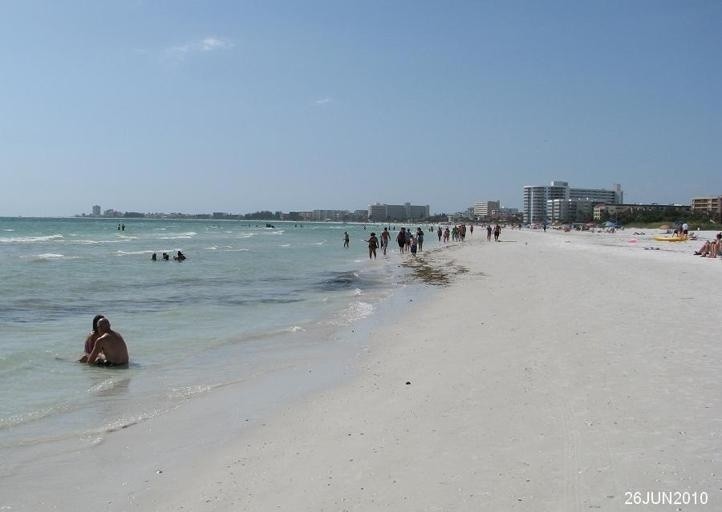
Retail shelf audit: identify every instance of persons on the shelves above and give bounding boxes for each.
[54,317,128,368]
[150,248,188,263]
[122,224,126,231]
[667,221,722,260]
[117,224,121,230]
[84,315,106,356]
[340,223,502,263]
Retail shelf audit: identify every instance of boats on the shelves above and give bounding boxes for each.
[654,231,695,241]
